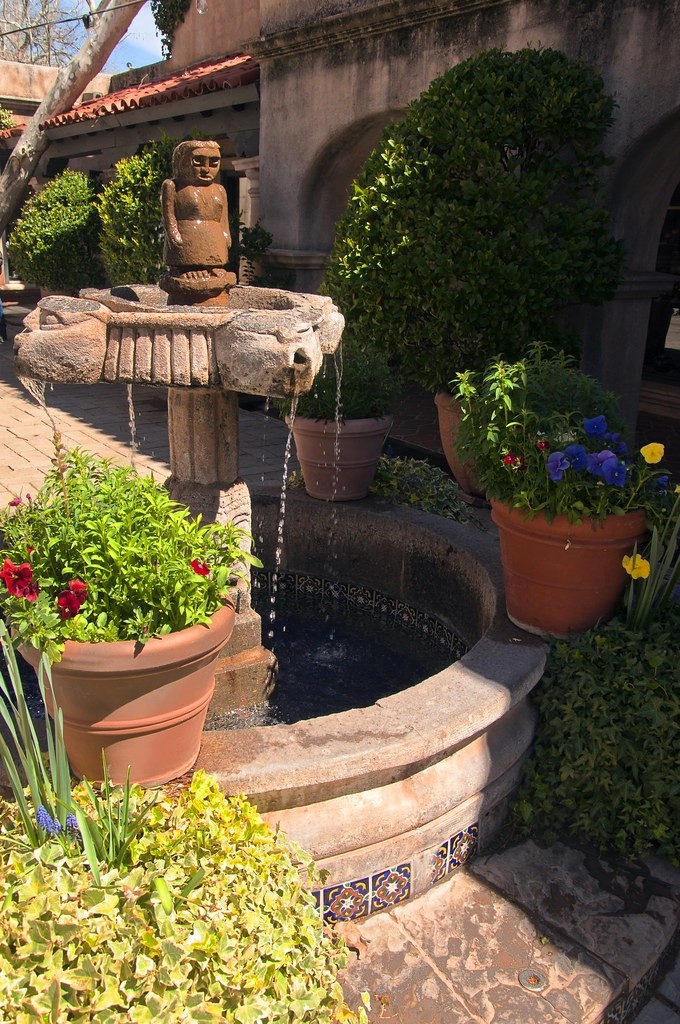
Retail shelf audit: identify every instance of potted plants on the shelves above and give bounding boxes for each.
[391,285,516,499]
[277,355,395,501]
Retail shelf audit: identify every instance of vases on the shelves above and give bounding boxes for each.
[12,595,235,792]
[490,498,645,641]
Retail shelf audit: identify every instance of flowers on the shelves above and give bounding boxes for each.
[0,438,264,672]
[450,354,680,578]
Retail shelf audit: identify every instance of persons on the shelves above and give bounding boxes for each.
[160,140,232,280]
